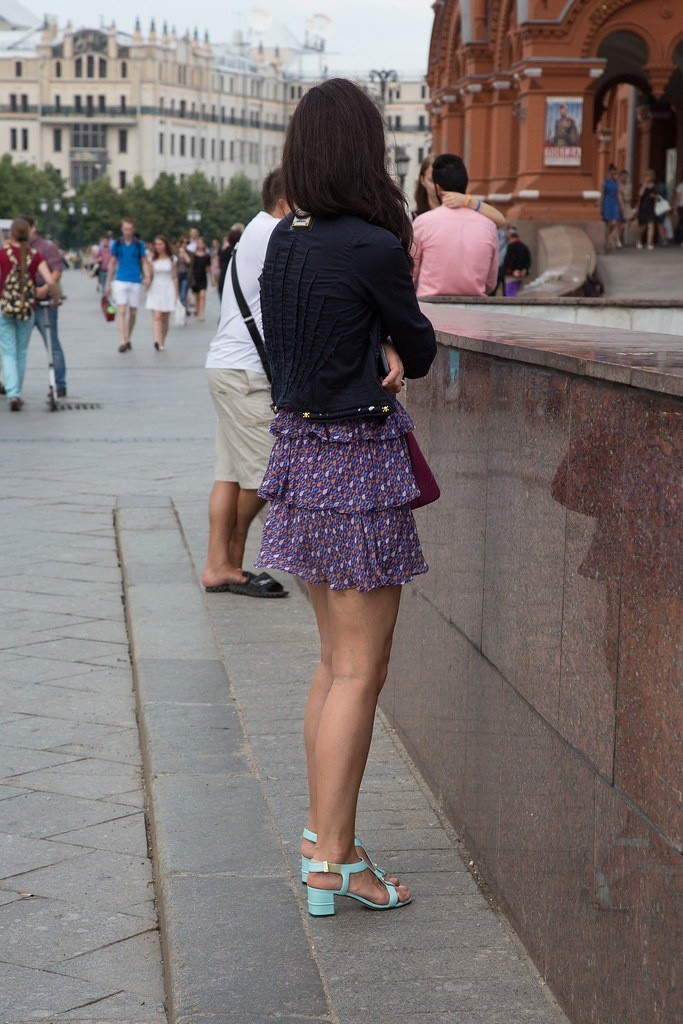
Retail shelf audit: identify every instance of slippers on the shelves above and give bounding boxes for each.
[204,566,292,599]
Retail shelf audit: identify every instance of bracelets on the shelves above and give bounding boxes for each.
[464,193,471,206]
[475,200,481,211]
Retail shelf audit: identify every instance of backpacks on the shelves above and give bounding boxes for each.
[1,242,37,322]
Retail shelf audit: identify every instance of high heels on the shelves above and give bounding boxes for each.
[299,824,397,887]
[307,844,415,916]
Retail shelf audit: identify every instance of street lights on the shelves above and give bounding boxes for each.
[40,197,61,239]
[66,200,89,257]
[185,196,203,245]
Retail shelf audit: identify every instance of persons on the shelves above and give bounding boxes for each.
[256,80,440,920]
[0,215,247,412]
[410,151,532,298]
[202,168,296,599]
[601,163,683,249]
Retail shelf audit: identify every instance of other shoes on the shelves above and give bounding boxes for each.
[638,242,643,248]
[119,341,131,353]
[647,244,654,250]
[10,395,20,411]
[154,341,164,351]
[48,383,67,399]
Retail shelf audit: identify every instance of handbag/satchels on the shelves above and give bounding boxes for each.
[170,298,186,326]
[654,194,671,217]
[664,217,673,239]
[101,293,117,322]
[402,432,441,511]
